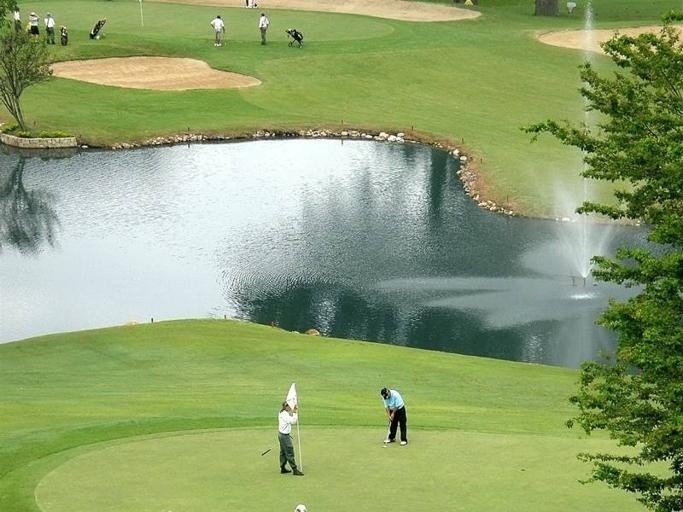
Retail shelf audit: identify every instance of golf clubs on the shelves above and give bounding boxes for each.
[381,421,391,448]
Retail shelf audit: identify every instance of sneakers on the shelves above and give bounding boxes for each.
[280,468,304,475]
[383,438,407,445]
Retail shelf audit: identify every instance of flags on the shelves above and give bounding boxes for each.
[285,382,298,411]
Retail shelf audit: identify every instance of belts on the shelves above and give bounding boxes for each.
[278,431,293,439]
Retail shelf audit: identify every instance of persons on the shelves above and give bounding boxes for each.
[277,401,304,477]
[380,387,409,446]
[43,13,55,44]
[257,12,269,45]
[13,7,22,35]
[210,14,225,48]
[26,11,40,43]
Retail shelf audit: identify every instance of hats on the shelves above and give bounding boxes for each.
[381,387,388,400]
